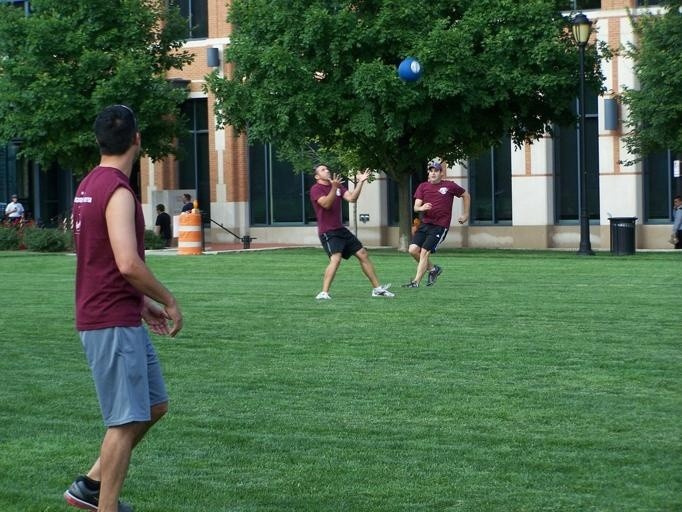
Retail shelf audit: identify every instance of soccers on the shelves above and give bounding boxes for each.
[398,57,421,82]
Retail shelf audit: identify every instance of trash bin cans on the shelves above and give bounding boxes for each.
[609,217,637,254]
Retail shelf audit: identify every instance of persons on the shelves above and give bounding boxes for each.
[400,162,472,289]
[5,193,26,224]
[671,195,682,249]
[181,193,194,212]
[309,164,395,300]
[62,105,184,512]
[153,204,172,246]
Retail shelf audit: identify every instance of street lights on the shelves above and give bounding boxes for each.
[569,9,596,254]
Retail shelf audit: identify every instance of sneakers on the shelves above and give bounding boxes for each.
[62,476,131,512]
[372,287,395,297]
[426,265,442,286]
[316,291,331,299]
[401,281,419,287]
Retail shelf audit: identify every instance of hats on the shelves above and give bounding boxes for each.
[429,162,441,170]
